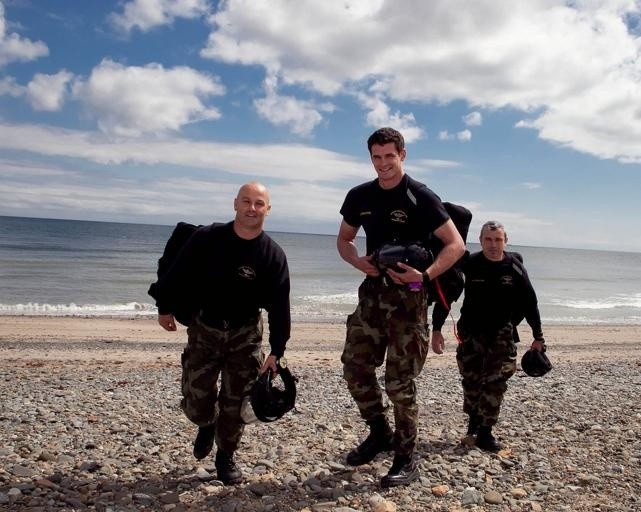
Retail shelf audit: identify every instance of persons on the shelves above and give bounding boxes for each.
[431,219,547,452]
[337,129,466,489]
[155,182,292,486]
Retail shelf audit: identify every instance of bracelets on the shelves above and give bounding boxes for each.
[535,337,545,342]
[422,271,431,287]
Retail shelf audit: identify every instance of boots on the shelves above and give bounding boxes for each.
[467,417,482,433]
[193,425,215,459]
[381,453,420,487]
[477,425,501,452]
[347,421,394,466]
[215,449,242,485]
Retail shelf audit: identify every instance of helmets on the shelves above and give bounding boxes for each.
[239,363,297,425]
[521,349,552,377]
[376,238,434,279]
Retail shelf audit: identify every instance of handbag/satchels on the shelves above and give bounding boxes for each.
[146,222,199,326]
[428,199,472,305]
